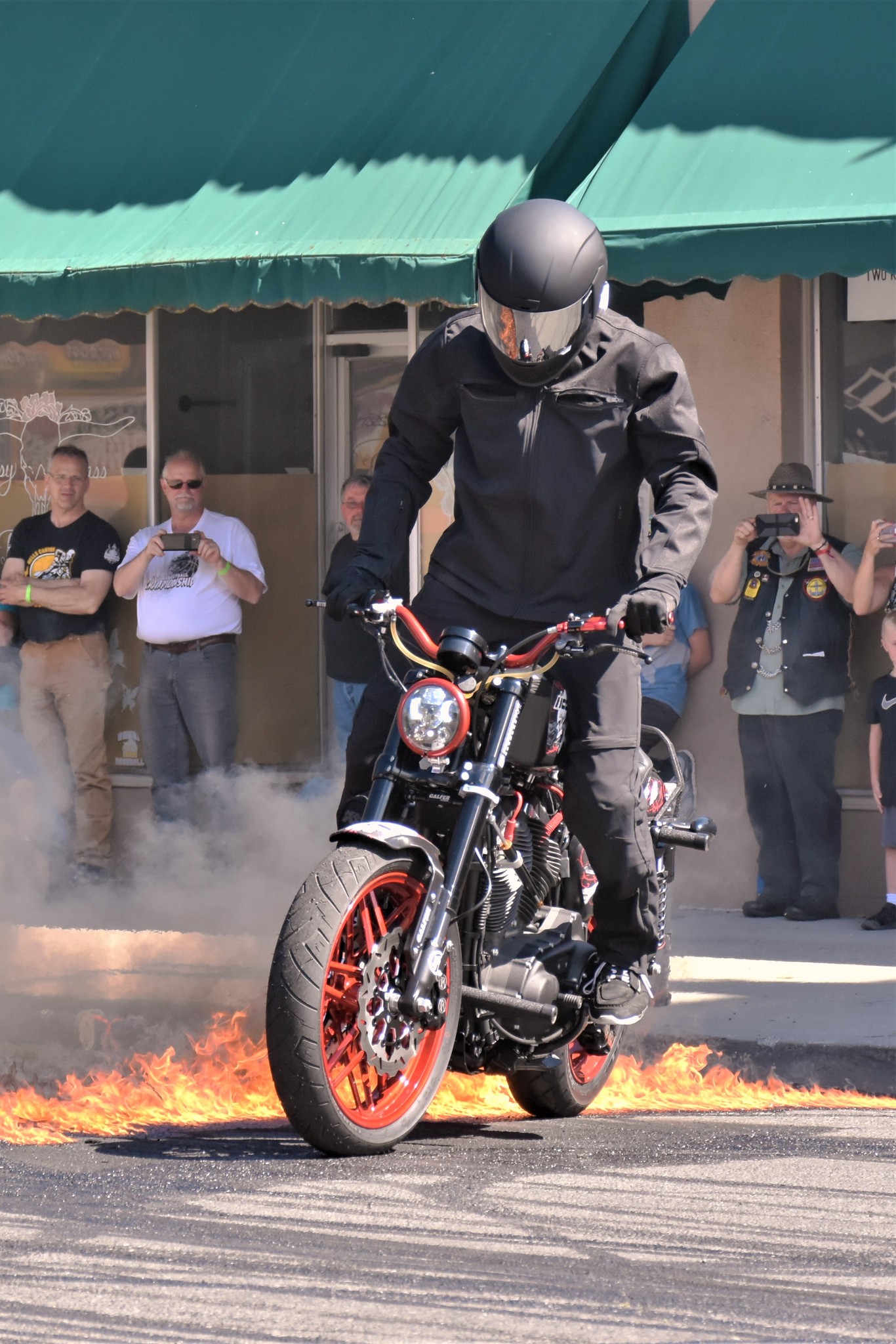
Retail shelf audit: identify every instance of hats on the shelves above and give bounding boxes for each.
[748,463,835,505]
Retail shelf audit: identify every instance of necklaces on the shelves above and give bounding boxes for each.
[764,550,810,577]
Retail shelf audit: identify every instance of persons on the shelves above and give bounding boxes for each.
[321,473,409,751]
[0,446,120,885]
[861,610,896,930]
[329,198,721,1025]
[852,518,896,616]
[708,460,864,921]
[641,582,712,823]
[114,448,268,868]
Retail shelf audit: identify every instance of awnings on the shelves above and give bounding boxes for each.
[0,0,896,323]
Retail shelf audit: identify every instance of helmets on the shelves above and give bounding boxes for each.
[475,198,608,385]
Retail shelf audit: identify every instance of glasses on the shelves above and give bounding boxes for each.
[342,499,366,510]
[47,471,89,486]
[164,476,204,489]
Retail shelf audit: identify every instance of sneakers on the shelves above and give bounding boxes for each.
[583,952,652,1027]
[860,902,896,930]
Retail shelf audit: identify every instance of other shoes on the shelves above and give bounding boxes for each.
[742,892,786,917]
[74,865,101,888]
[784,895,840,921]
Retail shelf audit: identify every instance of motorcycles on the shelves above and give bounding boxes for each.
[261,593,721,1157]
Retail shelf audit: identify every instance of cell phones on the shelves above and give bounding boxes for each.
[878,522,896,543]
[754,513,800,537]
[159,533,199,550]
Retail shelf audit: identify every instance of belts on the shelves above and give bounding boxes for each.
[144,634,237,653]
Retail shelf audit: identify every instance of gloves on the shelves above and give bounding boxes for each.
[608,588,676,644]
[323,568,382,623]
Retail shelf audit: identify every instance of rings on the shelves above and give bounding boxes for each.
[877,537,879,540]
[809,516,813,519]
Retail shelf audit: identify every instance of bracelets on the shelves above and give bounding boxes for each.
[217,561,230,575]
[26,584,32,604]
[814,539,835,559]
